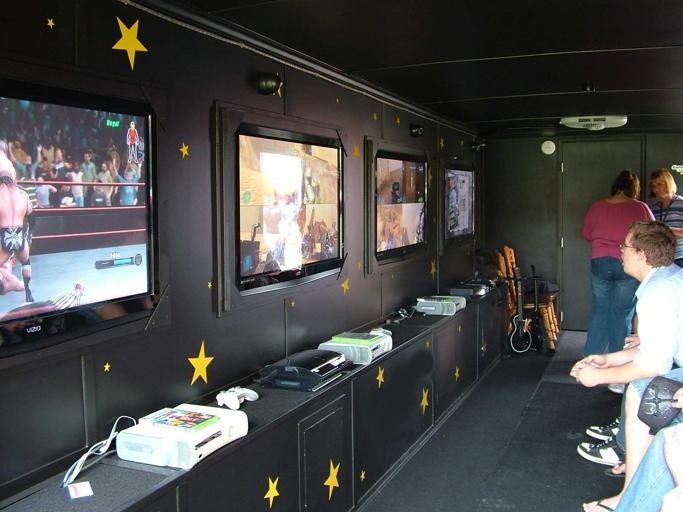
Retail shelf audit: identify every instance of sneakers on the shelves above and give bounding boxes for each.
[606,382,626,394]
[584,415,621,440]
[575,434,626,467]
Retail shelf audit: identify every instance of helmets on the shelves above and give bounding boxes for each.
[0,160,17,182]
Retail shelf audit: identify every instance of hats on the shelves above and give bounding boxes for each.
[637,377,683,435]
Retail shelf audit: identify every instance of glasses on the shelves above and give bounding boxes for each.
[619,241,636,249]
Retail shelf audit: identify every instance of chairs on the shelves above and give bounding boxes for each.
[495,245,559,353]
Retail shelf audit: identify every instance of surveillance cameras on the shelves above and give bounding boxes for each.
[257,72,281,94]
[411,126,424,137]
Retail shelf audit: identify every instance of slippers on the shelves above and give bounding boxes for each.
[595,498,613,512]
[603,463,625,478]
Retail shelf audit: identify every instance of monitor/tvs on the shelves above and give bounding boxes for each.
[234,122,344,291]
[374,149,428,261]
[444,163,476,244]
[0,78,159,359]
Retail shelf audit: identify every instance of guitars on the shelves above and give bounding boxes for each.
[508,265,550,354]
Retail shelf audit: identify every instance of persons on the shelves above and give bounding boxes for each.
[0,97,148,301]
[382,211,409,251]
[262,222,335,276]
[568,216,683,512]
[646,167,683,268]
[447,172,459,231]
[579,169,658,359]
